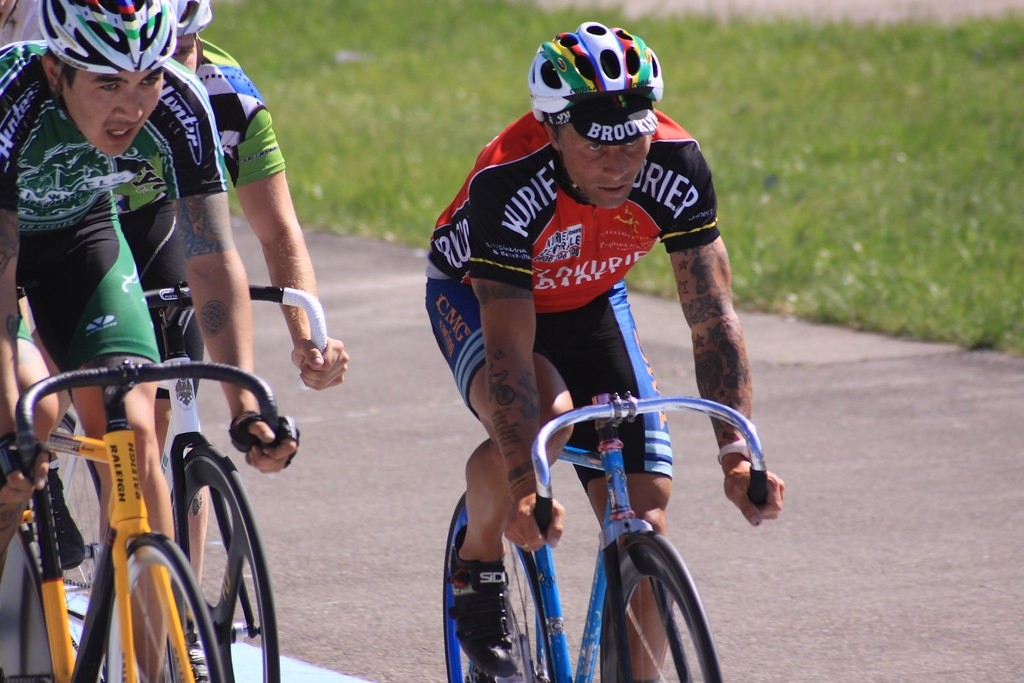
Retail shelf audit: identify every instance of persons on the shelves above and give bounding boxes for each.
[426,22,782,683]
[0,1,349,683]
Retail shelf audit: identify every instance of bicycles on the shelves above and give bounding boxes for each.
[1,285,328,683]
[440,395,769,683]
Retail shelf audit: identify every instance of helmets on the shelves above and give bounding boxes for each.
[528,21,663,145]
[176,0,213,36]
[37,0,177,74]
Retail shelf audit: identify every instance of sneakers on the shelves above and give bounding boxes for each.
[449,525,520,677]
[47,467,86,569]
[185,633,210,683]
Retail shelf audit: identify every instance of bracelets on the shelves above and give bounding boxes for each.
[717,440,752,463]
